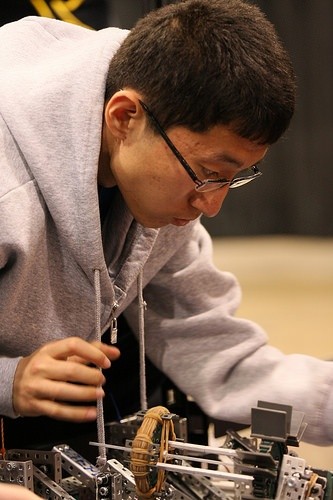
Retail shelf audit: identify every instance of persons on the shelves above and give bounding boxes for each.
[0,0,333,459]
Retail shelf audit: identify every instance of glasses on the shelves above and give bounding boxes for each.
[118,87,263,194]
